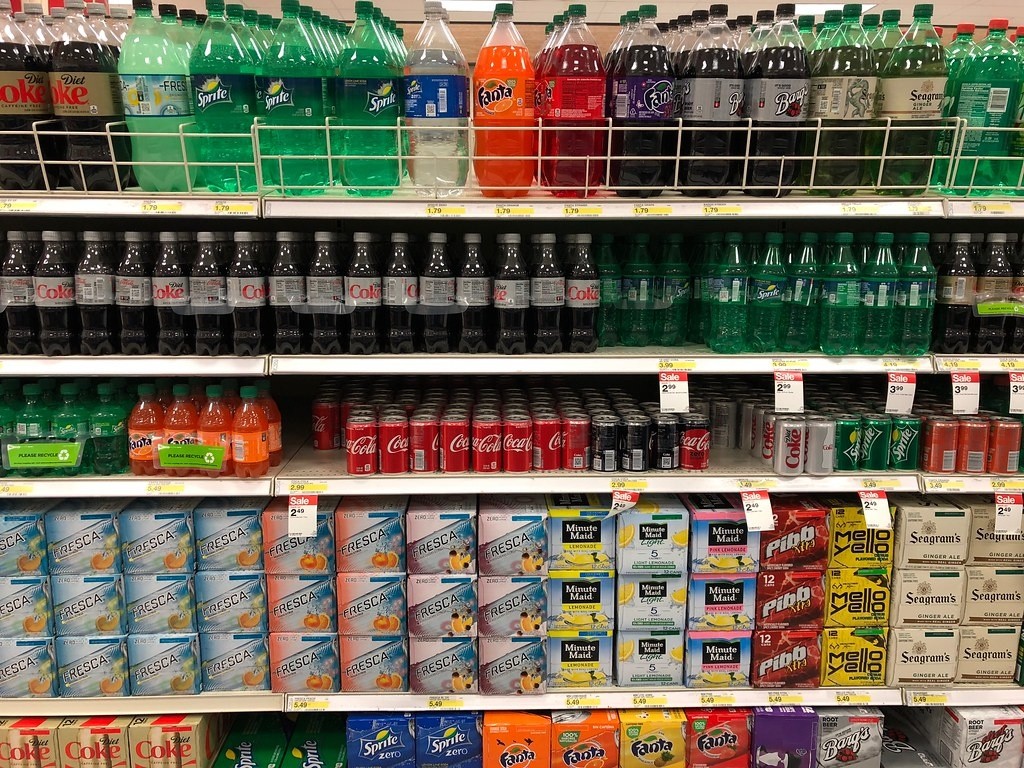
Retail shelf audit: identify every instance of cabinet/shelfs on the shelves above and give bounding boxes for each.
[0,195,1024,716]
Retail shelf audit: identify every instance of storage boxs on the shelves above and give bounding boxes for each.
[0,492,1023,700]
[0,704,1024,768]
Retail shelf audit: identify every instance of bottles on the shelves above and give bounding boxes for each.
[797,4,949,197]
[902,18,1024,197]
[403,0,470,197]
[473,3,536,198]
[0,230,602,356]
[189,0,408,198]
[932,233,1024,355]
[0,0,129,191]
[656,4,811,198]
[117,0,206,193]
[125,377,283,478]
[602,5,675,199]
[595,232,936,354]
[0,377,139,478]
[532,4,607,199]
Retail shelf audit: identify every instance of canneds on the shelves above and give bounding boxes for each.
[313,374,1024,477]
